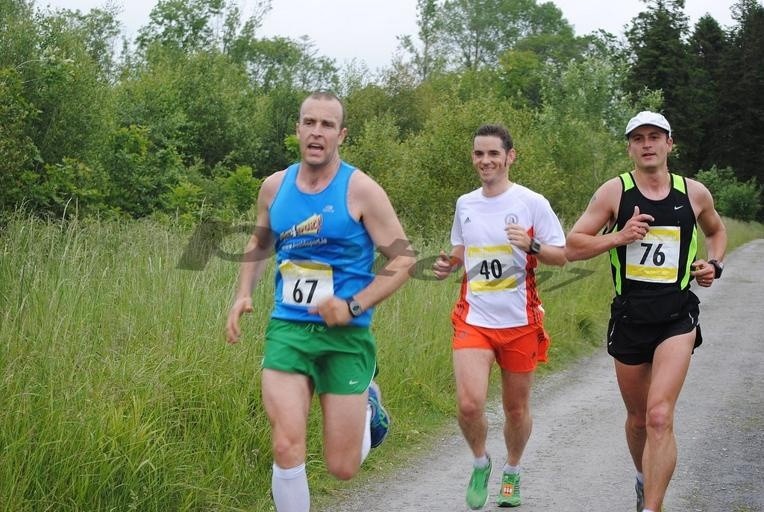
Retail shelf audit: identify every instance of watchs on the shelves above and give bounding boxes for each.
[526,237,540,255]
[708,260,724,279]
[345,296,362,317]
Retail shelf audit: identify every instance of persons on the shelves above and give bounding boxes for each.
[565,111,727,512]
[433,125,568,510]
[225,92,417,512]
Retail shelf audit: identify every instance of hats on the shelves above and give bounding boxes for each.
[625,111,671,137]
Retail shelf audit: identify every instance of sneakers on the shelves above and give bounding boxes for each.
[368,381,390,448]
[635,477,663,512]
[496,461,521,507]
[467,456,492,510]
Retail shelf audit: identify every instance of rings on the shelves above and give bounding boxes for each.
[702,280,705,283]
